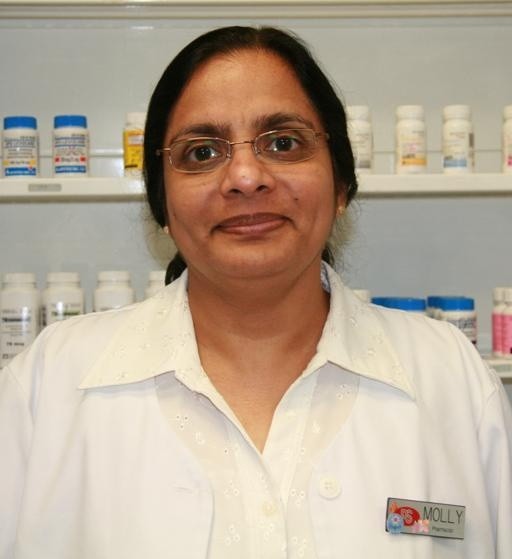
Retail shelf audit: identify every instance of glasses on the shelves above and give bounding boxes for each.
[151,125,331,176]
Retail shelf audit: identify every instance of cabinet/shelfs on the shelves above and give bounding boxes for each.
[0,173,510,381]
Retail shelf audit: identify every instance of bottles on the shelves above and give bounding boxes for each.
[370,296,478,350]
[3,115,40,177]
[145,270,168,301]
[51,114,89,177]
[1,271,41,366]
[42,271,86,329]
[441,104,475,175]
[490,286,512,361]
[345,105,376,175]
[502,106,511,172]
[393,105,427,175]
[93,270,133,312]
[121,111,146,180]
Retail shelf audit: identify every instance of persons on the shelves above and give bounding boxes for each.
[0,24,511,559]
